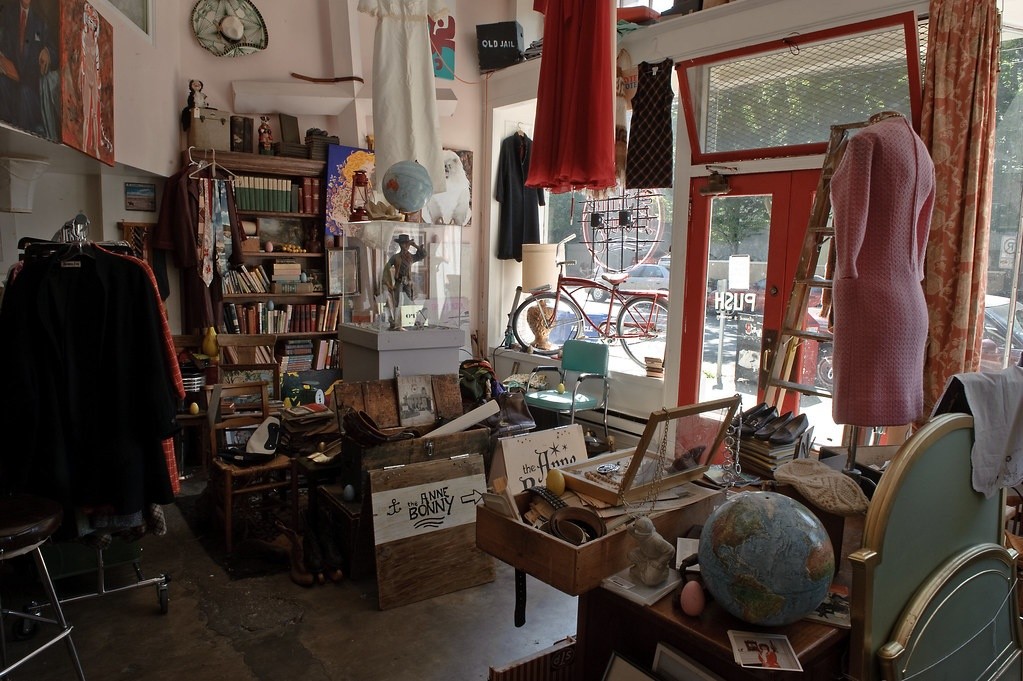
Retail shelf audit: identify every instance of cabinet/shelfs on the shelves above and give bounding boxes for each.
[181,148,344,408]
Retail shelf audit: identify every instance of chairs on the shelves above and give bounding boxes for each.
[524,339,610,438]
[203,380,299,557]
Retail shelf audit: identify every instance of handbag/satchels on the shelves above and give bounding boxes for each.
[479,387,536,436]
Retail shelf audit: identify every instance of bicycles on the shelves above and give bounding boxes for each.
[512,235,668,371]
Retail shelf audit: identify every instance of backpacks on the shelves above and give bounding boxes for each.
[458,357,497,403]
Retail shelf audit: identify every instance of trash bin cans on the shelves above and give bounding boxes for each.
[735,313,763,382]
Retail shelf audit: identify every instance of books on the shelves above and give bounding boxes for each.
[208,176,342,371]
[728,433,807,478]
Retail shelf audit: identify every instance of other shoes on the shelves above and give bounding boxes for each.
[769,413,808,445]
[728,401,768,429]
[737,406,779,436]
[754,410,794,440]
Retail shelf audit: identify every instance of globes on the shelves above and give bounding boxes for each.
[698,491,836,626]
[382,160,433,222]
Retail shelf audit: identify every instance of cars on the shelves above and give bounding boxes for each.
[708,272,826,328]
[815,294,1023,391]
[584,264,671,303]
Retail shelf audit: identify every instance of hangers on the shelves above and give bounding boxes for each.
[517,122,525,137]
[627,36,681,76]
[165,146,239,181]
[18,217,135,265]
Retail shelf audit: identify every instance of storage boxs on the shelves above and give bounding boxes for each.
[475,393,921,681]
[305,135,340,161]
[188,107,230,152]
[273,142,310,158]
[617,5,661,22]
[476,478,728,597]
[475,21,525,73]
[315,417,494,612]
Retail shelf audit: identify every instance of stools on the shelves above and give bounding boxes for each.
[0,492,86,681]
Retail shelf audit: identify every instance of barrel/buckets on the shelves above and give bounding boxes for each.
[175,360,208,414]
[523,243,556,293]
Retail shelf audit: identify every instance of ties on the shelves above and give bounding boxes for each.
[196,178,233,288]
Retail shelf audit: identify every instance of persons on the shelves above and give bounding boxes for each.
[756,643,780,668]
[383,234,427,330]
[830,110,936,426]
[628,516,675,586]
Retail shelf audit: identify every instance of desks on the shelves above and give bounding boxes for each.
[577,492,866,681]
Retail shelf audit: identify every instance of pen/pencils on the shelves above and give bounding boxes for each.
[419,310,426,320]
[807,433,810,453]
[809,436,816,451]
[802,442,806,459]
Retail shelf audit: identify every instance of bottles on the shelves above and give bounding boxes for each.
[287,474,309,511]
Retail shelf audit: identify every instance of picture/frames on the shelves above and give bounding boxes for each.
[124,182,156,211]
[384,230,427,267]
[325,246,361,298]
[382,269,430,300]
[218,363,281,401]
